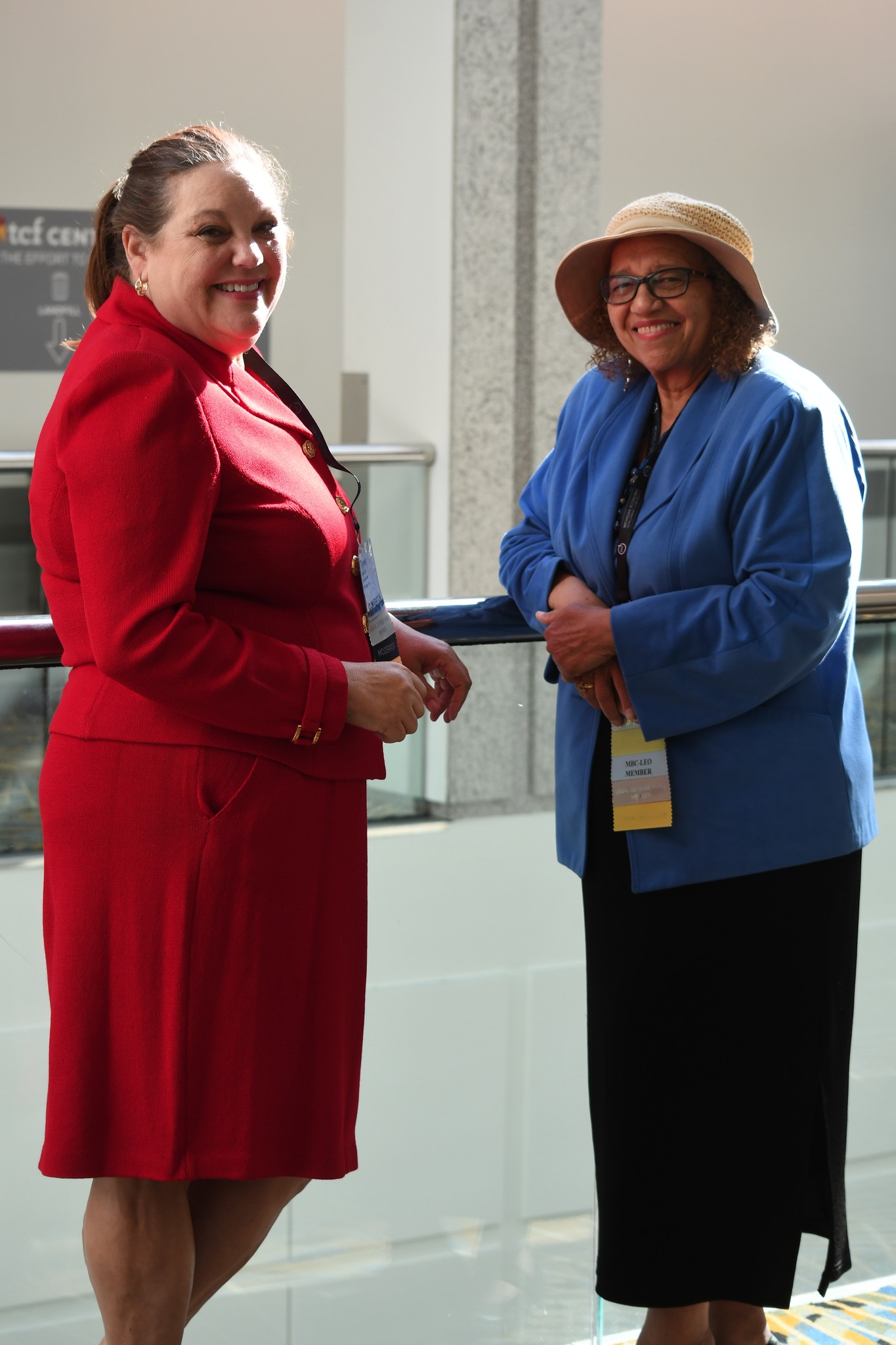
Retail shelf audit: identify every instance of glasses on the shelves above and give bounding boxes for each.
[599,267,722,305]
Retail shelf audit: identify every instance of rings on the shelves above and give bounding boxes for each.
[441,677,447,680]
[578,681,595,693]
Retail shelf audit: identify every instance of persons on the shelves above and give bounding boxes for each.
[26,119,471,1345]
[500,192,880,1342]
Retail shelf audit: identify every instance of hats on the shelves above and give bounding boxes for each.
[554,191,779,351]
